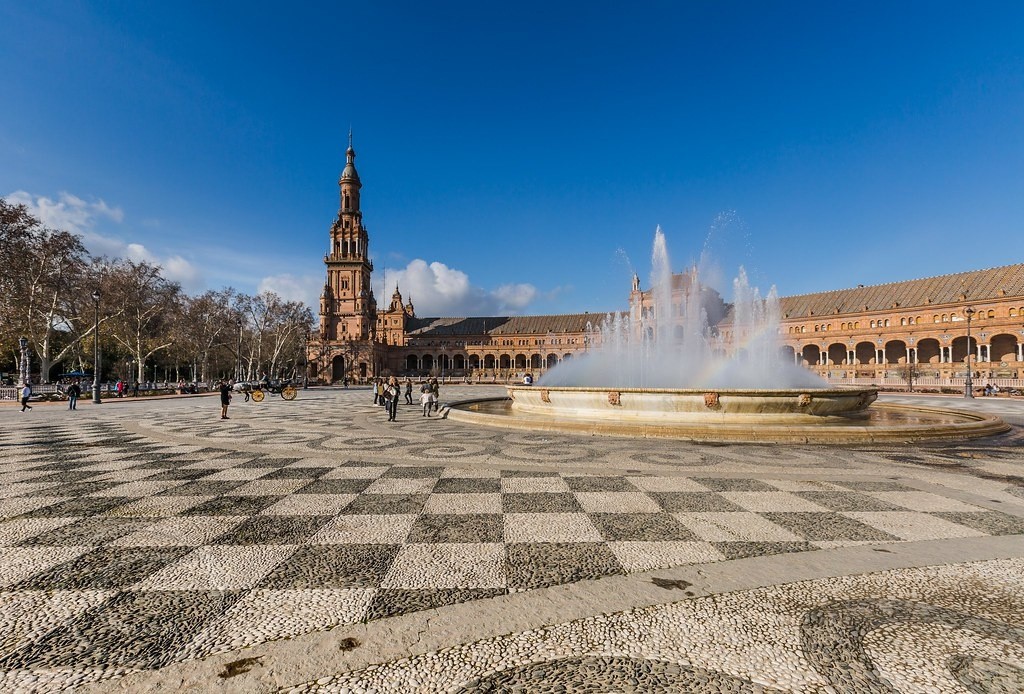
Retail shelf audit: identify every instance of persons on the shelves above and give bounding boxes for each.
[373,374,400,421]
[144,377,234,394]
[521,372,532,386]
[418,375,439,417]
[404,377,413,404]
[985,382,1000,396]
[114,379,139,397]
[19,383,32,413]
[61,377,93,410]
[219,378,232,418]
[260,371,268,381]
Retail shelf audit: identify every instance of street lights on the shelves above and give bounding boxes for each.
[16,334,29,402]
[132,360,136,383]
[583,334,588,353]
[303,334,308,388]
[125,362,129,382]
[236,320,242,383]
[964,308,974,398]
[154,364,157,383]
[91,290,102,403]
[538,344,543,377]
[441,344,446,385]
[189,364,192,382]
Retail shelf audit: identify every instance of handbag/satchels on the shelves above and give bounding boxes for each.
[382,385,393,401]
[229,395,233,400]
[69,390,74,397]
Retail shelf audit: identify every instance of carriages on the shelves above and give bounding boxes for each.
[228,362,298,402]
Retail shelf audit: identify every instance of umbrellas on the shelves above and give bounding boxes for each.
[58,372,91,377]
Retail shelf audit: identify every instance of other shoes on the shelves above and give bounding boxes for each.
[388,417,392,421]
[29,407,32,411]
[392,419,397,422]
[220,416,230,419]
[19,410,24,413]
